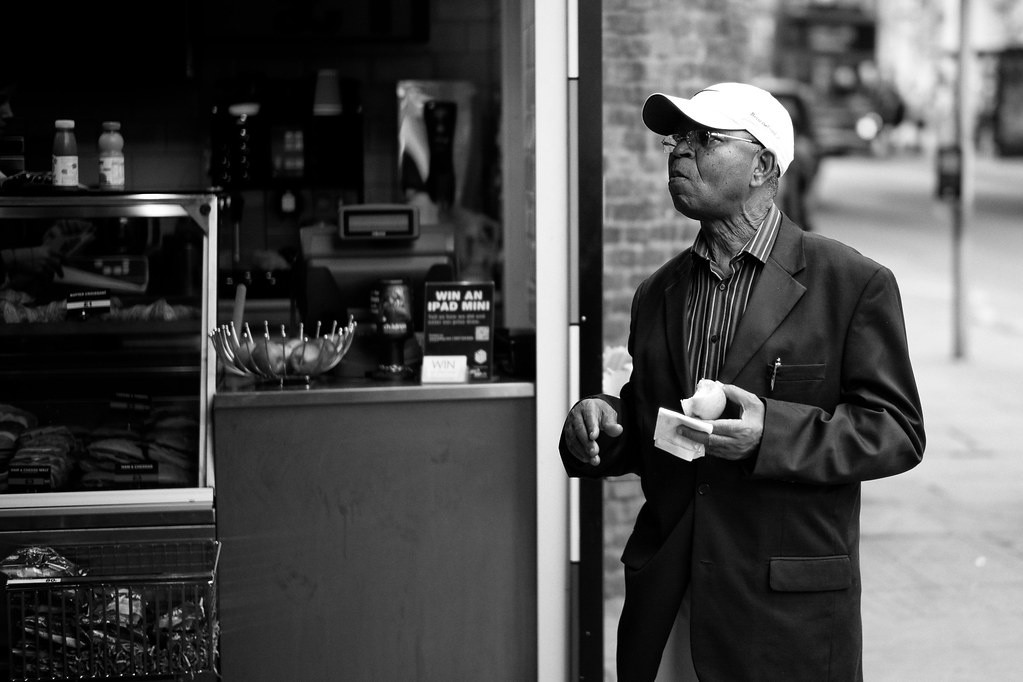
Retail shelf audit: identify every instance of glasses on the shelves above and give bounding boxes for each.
[661,130,765,154]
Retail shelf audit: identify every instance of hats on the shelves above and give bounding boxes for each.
[641,82,794,178]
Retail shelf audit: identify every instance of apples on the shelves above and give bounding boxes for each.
[232,332,346,376]
[680,378,727,420]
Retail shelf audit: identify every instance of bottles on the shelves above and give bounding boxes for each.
[98,122,125,191]
[52,120,79,191]
[378,280,411,372]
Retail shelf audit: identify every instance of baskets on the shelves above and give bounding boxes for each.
[3,537,223,681]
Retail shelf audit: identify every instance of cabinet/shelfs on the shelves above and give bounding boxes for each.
[0,193,218,517]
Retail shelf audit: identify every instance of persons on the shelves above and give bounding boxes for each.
[0,171,155,307]
[559,82,926,682]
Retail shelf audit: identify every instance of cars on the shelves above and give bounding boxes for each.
[814,110,871,158]
